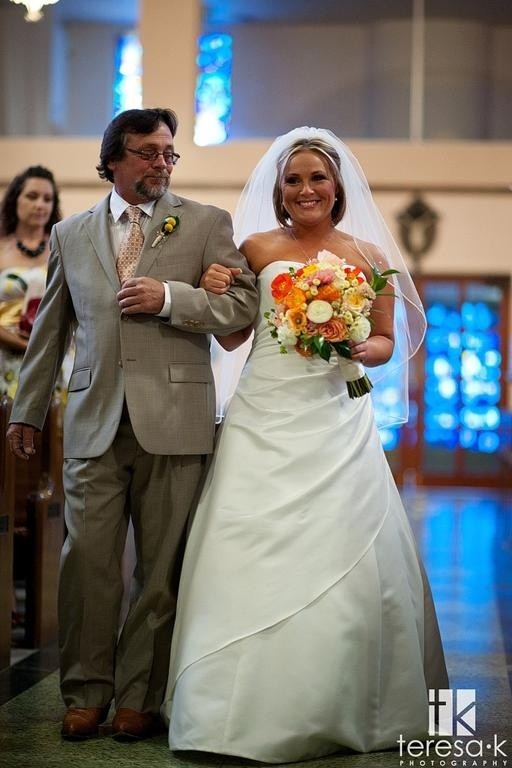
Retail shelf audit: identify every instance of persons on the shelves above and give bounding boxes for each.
[160,126,453,766]
[0,166,75,628]
[5,107,263,741]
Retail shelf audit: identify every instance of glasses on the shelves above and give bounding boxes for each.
[125,145,181,164]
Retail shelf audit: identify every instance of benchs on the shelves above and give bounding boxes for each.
[0,392,69,704]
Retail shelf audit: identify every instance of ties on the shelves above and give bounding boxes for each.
[116,204,146,289]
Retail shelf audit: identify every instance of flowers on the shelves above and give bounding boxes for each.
[262,248,402,401]
[151,213,180,249]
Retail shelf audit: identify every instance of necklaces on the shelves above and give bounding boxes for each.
[291,225,335,262]
[13,230,48,257]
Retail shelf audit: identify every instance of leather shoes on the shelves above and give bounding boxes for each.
[112,707,147,742]
[62,704,111,743]
